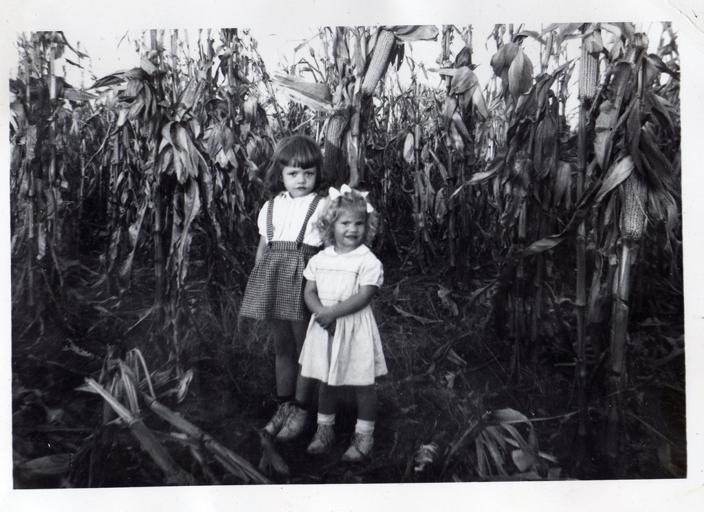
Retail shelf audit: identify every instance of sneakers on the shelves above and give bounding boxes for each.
[306,424,336,454]
[340,432,374,463]
[261,401,312,442]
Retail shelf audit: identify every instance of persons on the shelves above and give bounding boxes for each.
[297,182,390,461]
[237,137,329,441]
